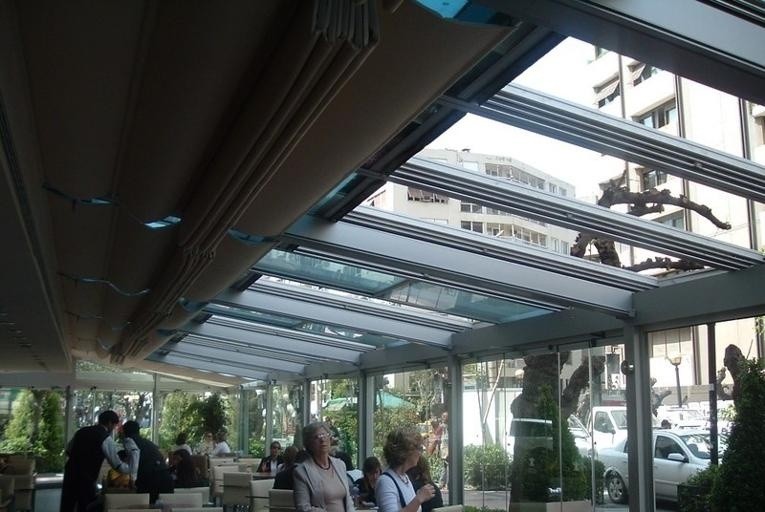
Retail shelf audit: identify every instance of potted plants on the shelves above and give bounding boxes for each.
[509,446,592,512]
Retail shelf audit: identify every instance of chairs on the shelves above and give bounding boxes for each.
[102,457,301,512]
[1,453,36,511]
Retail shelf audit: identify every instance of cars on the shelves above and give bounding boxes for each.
[502,403,730,506]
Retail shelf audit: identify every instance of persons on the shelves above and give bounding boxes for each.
[659,421,670,459]
